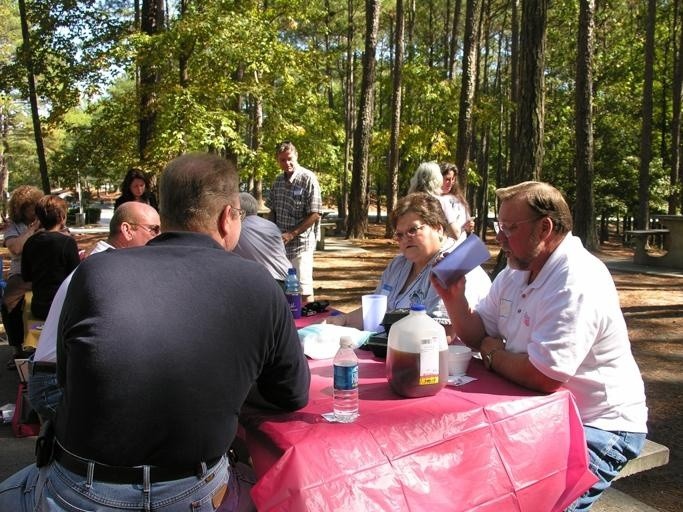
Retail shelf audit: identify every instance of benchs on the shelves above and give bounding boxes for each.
[610,439,670,481]
[21,289,43,351]
[315,223,337,251]
[627,228,668,235]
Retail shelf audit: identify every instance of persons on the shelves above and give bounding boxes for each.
[429,181,648,512]
[1,168,290,371]
[408,162,475,240]
[264,141,324,303]
[27,202,161,417]
[316,192,493,346]
[0,152,312,511]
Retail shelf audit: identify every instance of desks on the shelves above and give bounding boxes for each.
[633,216,683,268]
[319,211,335,221]
[237,297,600,512]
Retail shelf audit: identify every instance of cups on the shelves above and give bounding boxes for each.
[361,294,388,333]
[447,345,472,376]
[430,234,492,290]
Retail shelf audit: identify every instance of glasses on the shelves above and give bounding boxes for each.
[499,216,534,232]
[393,224,425,241]
[130,224,160,235]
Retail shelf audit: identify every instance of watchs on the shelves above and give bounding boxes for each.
[484,349,498,371]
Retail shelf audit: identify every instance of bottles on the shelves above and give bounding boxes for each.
[285,268,303,318]
[333,336,359,424]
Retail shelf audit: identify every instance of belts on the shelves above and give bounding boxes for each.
[53,440,221,483]
